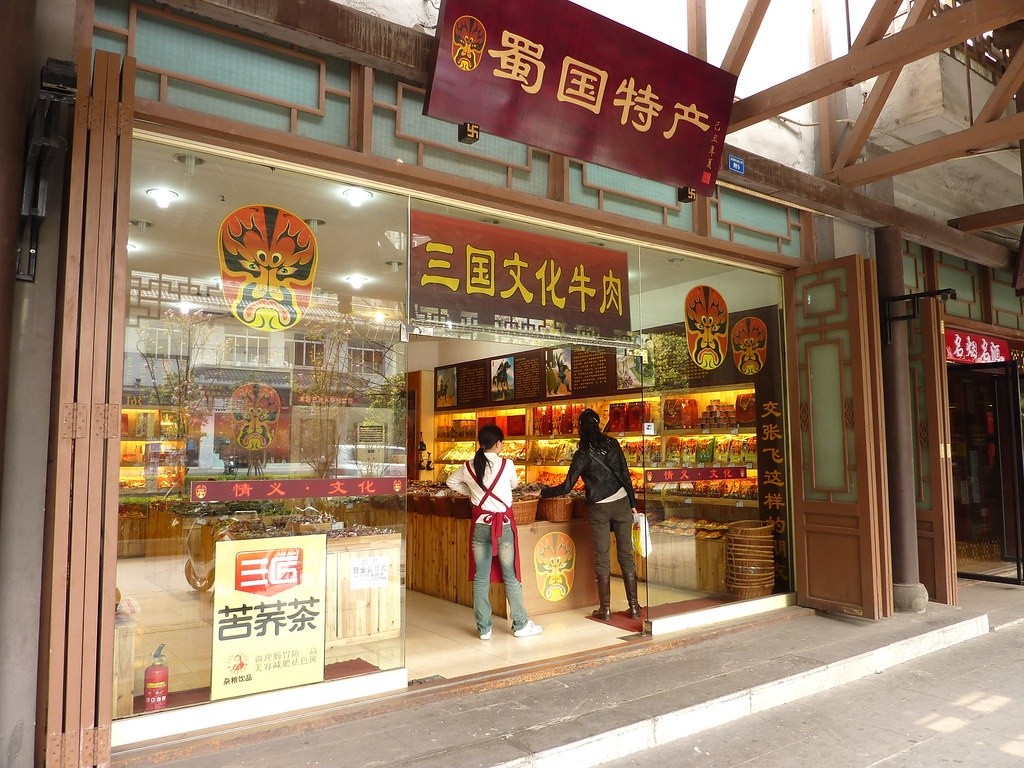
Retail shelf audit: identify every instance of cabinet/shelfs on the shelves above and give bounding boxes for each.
[433,381,763,596]
[119,405,189,494]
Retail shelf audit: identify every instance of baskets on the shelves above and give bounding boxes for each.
[574,498,589,518]
[723,519,776,601]
[539,496,573,522]
[369,492,472,519]
[511,498,539,524]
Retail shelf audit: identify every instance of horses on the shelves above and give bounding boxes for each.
[437,383,449,404]
[492,361,511,392]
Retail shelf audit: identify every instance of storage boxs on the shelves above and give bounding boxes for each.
[734,392,756,427]
[450,398,700,440]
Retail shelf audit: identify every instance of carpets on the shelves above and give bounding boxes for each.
[585,596,738,634]
[133,658,381,715]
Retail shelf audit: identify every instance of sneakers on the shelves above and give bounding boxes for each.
[481,627,492,639]
[514,619,543,637]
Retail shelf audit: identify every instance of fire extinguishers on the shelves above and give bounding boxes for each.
[145,642,169,711]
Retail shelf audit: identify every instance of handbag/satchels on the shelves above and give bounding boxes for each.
[631,511,652,557]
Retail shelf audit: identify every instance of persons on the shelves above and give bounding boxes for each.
[527,407,643,620]
[446,424,543,640]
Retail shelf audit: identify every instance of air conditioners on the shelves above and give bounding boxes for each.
[353,422,388,479]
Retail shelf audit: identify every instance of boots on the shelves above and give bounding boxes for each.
[593,575,611,620]
[623,572,642,619]
